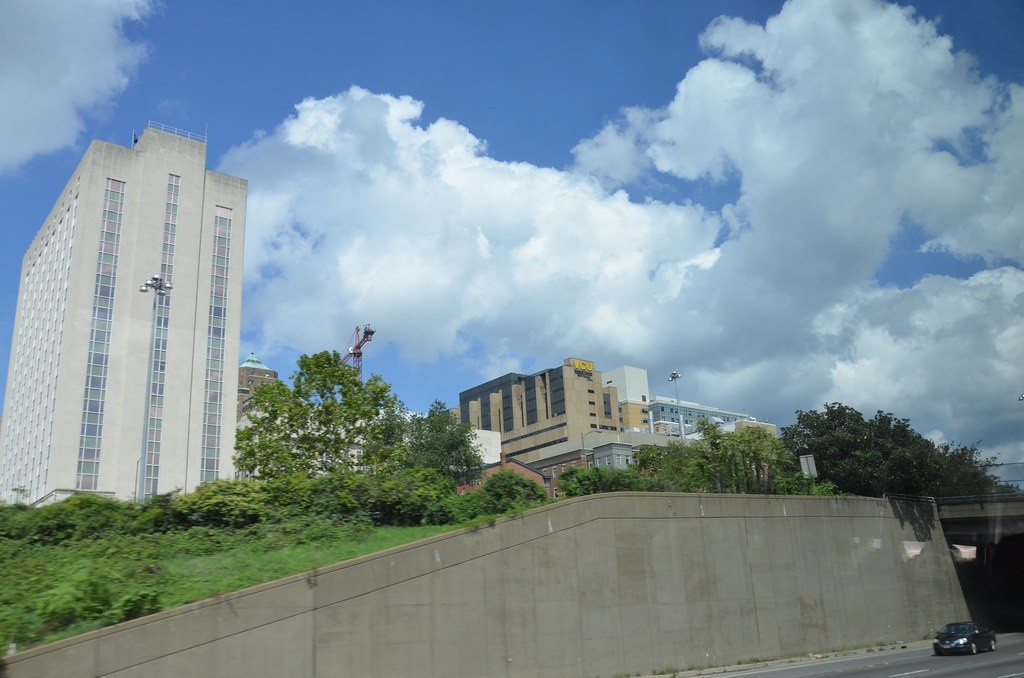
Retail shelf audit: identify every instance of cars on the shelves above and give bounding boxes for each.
[933,621,997,656]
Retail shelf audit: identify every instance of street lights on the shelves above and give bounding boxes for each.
[135,273,174,506]
[581,428,602,468]
[669,369,685,441]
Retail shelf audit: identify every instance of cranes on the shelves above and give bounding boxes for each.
[336,323,376,388]
